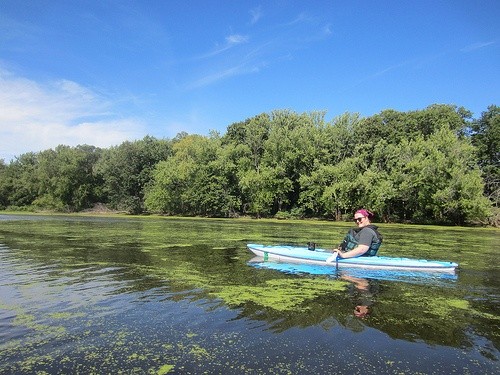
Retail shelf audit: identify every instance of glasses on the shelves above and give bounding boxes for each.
[353,217,365,222]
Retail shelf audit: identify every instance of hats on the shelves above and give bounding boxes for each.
[355,209,369,217]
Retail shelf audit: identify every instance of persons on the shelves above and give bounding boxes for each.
[333,210,382,259]
[337,275,383,320]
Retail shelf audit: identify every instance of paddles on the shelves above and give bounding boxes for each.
[324,244,343,266]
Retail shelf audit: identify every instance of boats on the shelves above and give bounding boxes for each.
[247,243,459,271]
